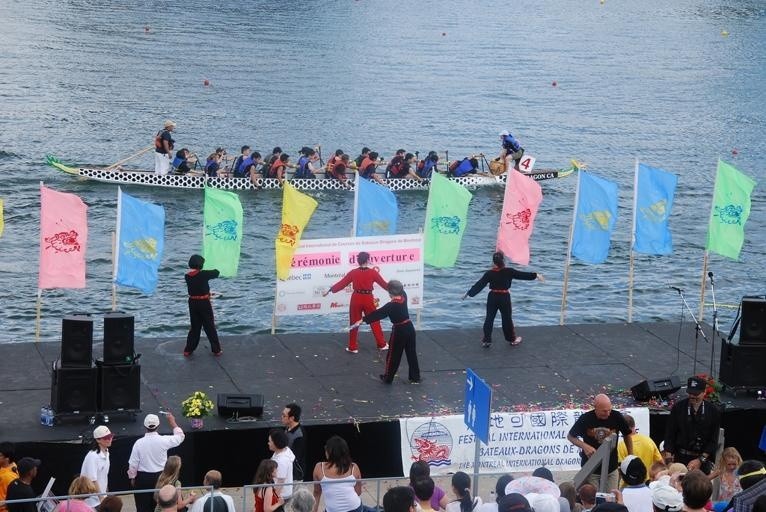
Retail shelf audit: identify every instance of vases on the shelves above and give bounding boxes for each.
[190,417,205,429]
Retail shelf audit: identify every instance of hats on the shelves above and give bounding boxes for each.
[165,119,175,127]
[93,426,115,439]
[388,280,402,295]
[143,414,159,430]
[498,467,570,512]
[617,455,645,480]
[16,458,41,473]
[686,376,705,394]
[499,130,510,136]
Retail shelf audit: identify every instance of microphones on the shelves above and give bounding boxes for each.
[708,272,715,285]
[669,285,681,294]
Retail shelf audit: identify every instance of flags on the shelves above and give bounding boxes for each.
[567,171,621,267]
[201,185,243,281]
[706,152,760,267]
[493,167,546,269]
[273,181,318,283]
[112,192,167,297]
[350,171,403,245]
[631,155,682,260]
[418,168,474,272]
[35,186,91,292]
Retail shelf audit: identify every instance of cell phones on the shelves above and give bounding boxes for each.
[595,492,616,507]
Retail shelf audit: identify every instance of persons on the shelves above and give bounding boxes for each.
[449,156,479,178]
[664,376,721,476]
[0,412,235,512]
[154,119,175,174]
[181,253,227,361]
[568,393,633,493]
[498,129,522,175]
[253,402,372,512]
[352,277,422,388]
[460,250,545,349]
[233,144,438,188]
[170,147,228,177]
[321,251,392,356]
[379,449,766,512]
[617,415,665,486]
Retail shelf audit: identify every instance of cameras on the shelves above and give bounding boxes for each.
[159,411,169,416]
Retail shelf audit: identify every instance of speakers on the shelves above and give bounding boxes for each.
[217,393,264,416]
[103,313,134,363]
[719,336,766,388]
[631,375,681,401]
[739,296,766,345]
[61,315,93,367]
[50,359,98,414]
[95,354,141,412]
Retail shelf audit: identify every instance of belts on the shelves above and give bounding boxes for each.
[191,295,208,299]
[679,449,702,456]
[490,289,508,293]
[393,319,410,325]
[353,290,371,293]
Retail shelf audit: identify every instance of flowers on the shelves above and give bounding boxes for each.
[179,390,216,416]
[694,373,724,404]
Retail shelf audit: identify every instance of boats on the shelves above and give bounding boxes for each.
[46,154,587,193]
